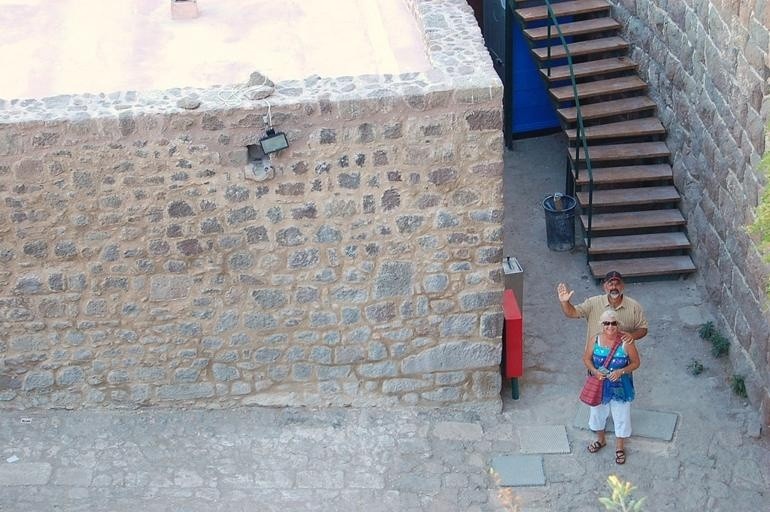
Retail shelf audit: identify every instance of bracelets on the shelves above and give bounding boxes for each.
[622,368,624,374]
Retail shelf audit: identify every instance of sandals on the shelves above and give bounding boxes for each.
[615,450,626,464]
[587,441,606,453]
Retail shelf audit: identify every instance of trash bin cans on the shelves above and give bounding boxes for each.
[542,195,577,251]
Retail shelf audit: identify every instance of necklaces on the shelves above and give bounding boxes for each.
[604,336,616,348]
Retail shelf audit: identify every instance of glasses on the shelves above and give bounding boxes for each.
[601,321,618,326]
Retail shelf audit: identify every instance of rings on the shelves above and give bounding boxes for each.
[600,377,602,381]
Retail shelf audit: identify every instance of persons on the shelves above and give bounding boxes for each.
[583,310,640,465]
[557,270,648,353]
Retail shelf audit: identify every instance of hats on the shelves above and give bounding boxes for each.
[603,271,622,284]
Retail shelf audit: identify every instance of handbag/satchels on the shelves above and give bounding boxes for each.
[579,376,604,406]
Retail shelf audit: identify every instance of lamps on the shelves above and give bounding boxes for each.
[259,128,290,156]
